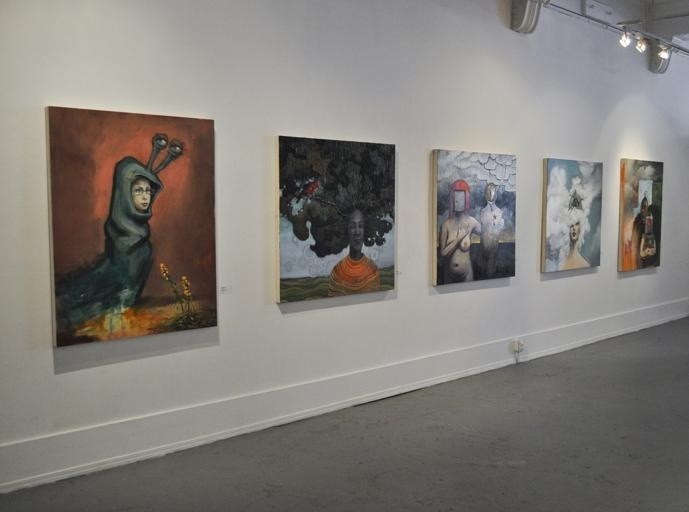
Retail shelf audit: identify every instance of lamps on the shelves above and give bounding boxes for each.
[618,23,673,61]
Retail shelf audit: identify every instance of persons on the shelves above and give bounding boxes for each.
[327,209,380,297]
[439,179,505,284]
[633,160,659,215]
[556,207,590,271]
[629,196,661,269]
[479,182,503,278]
[128,174,155,214]
